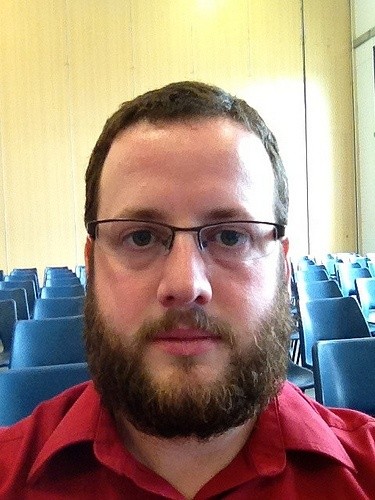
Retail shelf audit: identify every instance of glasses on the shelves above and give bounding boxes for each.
[83,219,286,261]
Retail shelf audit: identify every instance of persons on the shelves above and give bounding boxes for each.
[0,80,375,500]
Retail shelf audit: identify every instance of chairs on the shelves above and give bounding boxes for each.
[0,252,375,428]
